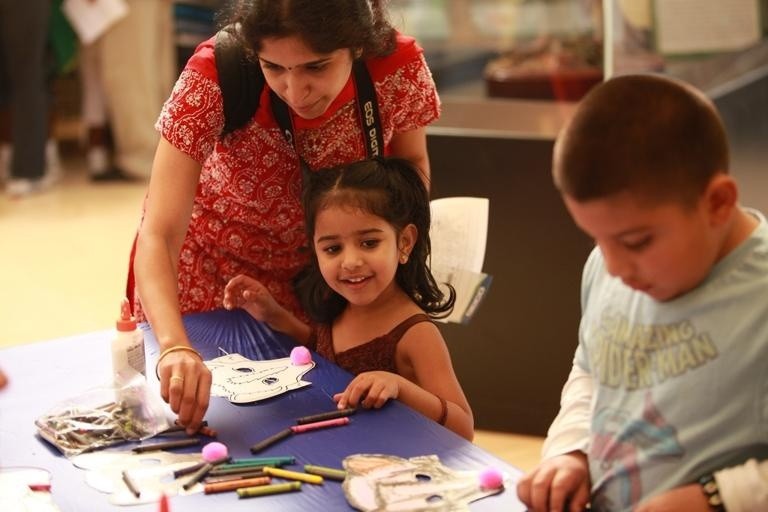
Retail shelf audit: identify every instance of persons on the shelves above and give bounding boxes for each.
[222,160,477,445]
[126,0,444,437]
[517,73,768,512]
[0,1,59,193]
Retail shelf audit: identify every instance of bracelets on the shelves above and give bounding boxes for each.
[697,473,727,512]
[155,345,204,382]
[434,393,448,426]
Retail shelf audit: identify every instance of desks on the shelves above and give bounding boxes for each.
[0,307,593,512]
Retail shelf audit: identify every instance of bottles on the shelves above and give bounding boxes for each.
[113,301,147,380]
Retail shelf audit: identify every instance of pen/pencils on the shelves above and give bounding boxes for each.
[122,407,356,512]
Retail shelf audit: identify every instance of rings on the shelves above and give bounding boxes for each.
[169,376,184,383]
